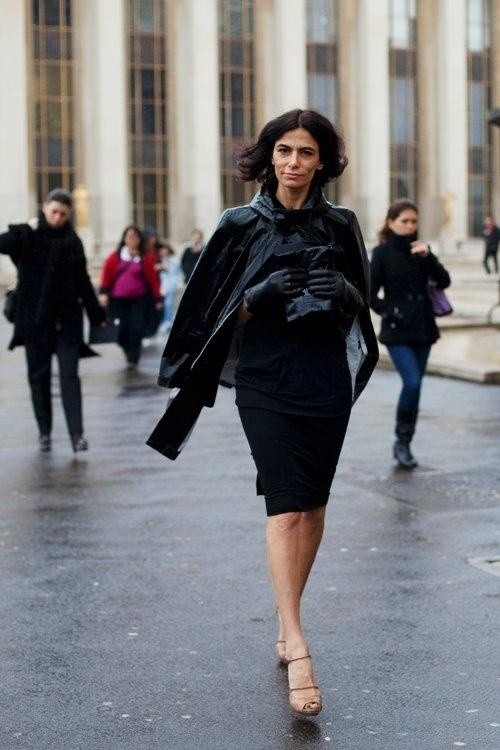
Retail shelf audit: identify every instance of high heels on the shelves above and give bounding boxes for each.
[276,605,322,716]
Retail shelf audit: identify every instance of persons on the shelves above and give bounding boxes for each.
[0,187,105,453]
[97,226,205,371]
[222,108,379,718]
[482,217,500,275]
[370,199,452,467]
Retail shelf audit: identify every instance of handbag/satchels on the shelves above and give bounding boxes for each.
[427,278,453,316]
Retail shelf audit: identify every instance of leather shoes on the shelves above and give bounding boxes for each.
[393,442,417,468]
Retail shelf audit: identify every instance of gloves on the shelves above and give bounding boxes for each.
[307,268,365,314]
[244,268,308,314]
